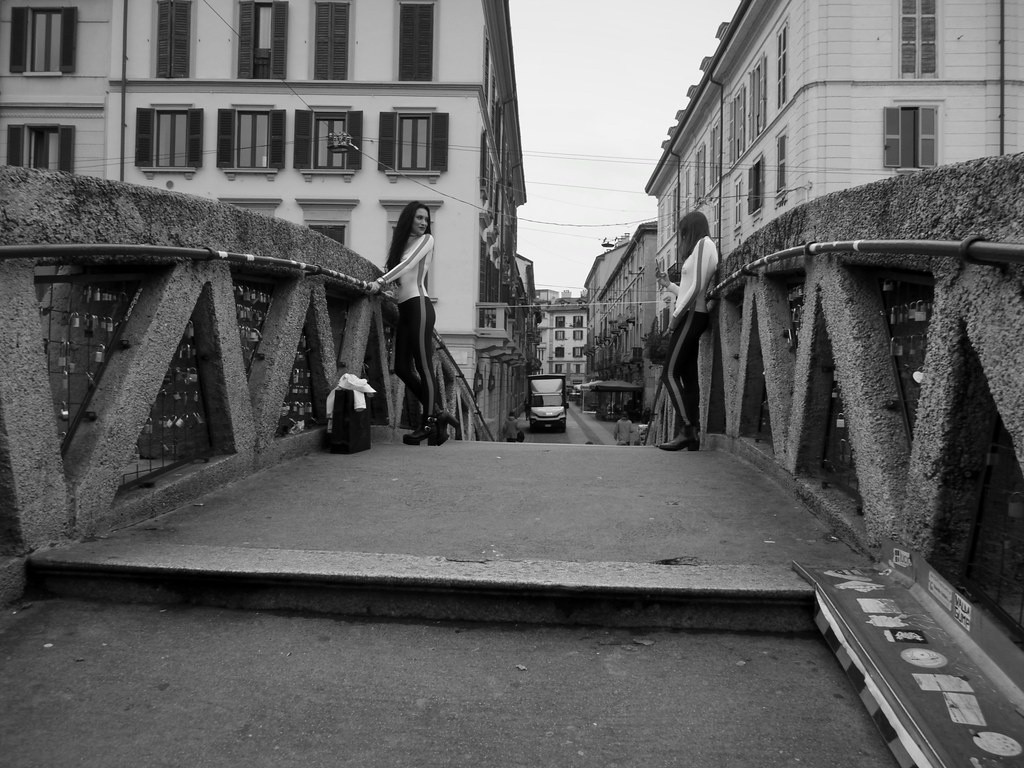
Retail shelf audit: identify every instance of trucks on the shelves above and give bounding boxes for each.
[525,373,569,433]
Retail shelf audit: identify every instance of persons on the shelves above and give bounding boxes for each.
[524,400,530,420]
[654,210,719,451]
[613,411,634,445]
[502,411,522,442]
[365,200,459,446]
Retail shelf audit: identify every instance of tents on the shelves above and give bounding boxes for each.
[574,380,603,414]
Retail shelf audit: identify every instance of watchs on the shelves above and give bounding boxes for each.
[376,277,385,286]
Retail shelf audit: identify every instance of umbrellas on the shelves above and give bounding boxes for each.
[590,379,642,421]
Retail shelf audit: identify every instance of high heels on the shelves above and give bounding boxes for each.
[428,411,459,446]
[402,413,437,446]
[660,425,701,451]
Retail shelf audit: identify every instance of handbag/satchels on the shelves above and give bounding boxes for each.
[517,431,524,442]
[330,386,372,455]
